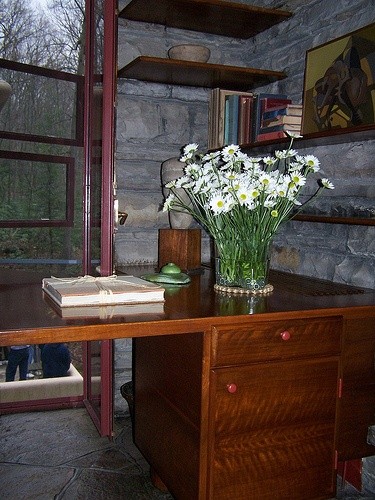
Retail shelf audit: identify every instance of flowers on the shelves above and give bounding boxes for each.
[161,143,334,286]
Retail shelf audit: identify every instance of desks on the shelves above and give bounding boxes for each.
[0,263,375,500]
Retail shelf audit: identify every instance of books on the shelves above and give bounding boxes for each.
[42,291,165,319]
[208,87,303,149]
[42,276,166,308]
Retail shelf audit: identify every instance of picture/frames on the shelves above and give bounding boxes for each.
[301,22,375,135]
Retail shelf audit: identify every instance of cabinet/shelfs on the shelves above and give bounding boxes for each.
[118,0,295,91]
[129,314,375,500]
[209,123,375,226]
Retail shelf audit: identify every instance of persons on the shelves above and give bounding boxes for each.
[41,342,71,379]
[6,346,30,382]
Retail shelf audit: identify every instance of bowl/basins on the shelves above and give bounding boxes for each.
[168,44,211,63]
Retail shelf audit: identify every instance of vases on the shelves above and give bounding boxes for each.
[215,237,273,291]
